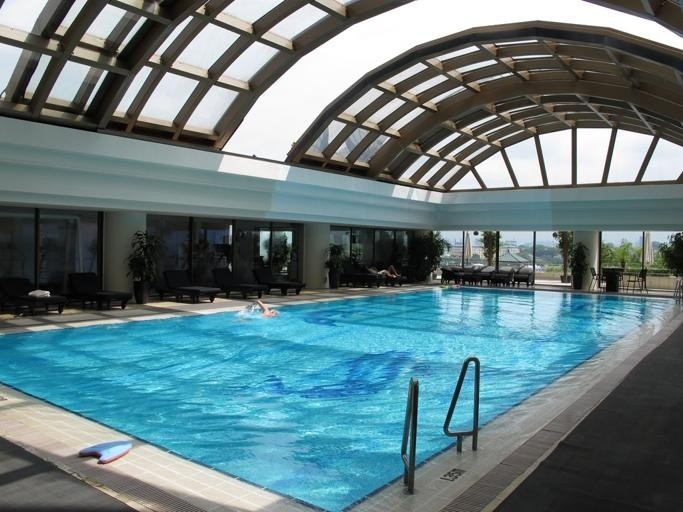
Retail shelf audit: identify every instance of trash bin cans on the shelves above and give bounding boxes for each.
[606,272,619,292]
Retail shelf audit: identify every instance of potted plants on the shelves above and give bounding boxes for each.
[322,242,345,290]
[568,241,590,290]
[552,231,575,283]
[123,230,164,304]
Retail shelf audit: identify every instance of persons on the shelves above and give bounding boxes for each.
[255,300,277,318]
[363,264,402,278]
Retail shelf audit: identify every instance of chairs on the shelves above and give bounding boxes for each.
[340,257,409,288]
[66,268,132,310]
[250,263,304,295]
[440,264,535,288]
[210,266,268,299]
[159,272,221,305]
[589,262,649,295]
[3,275,69,316]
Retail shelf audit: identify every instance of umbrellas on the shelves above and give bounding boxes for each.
[464,230,472,265]
[638,231,654,268]
